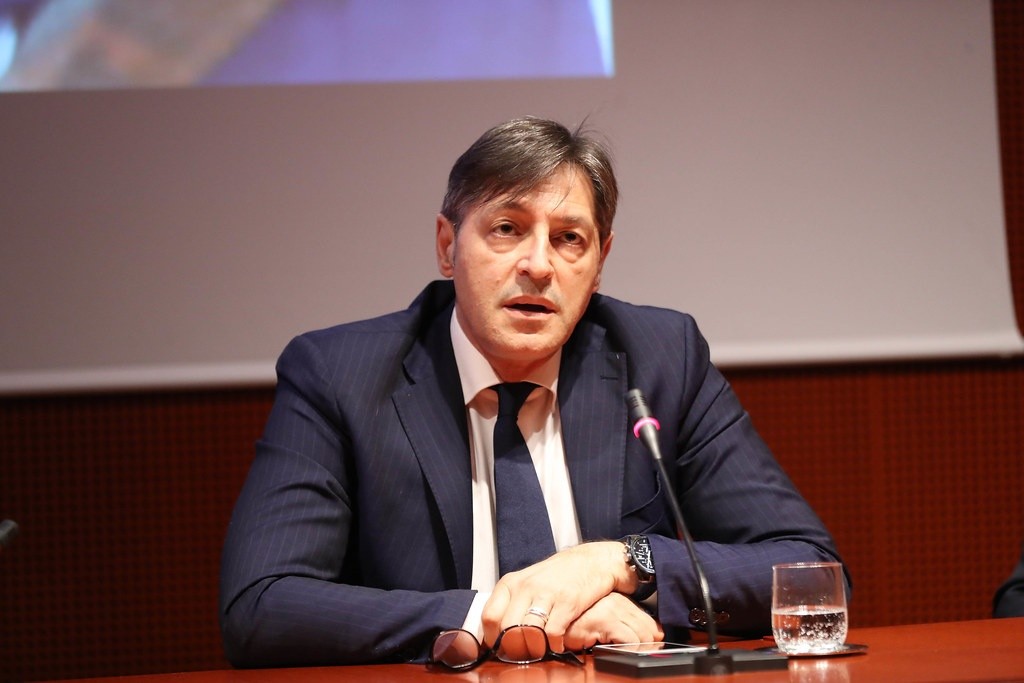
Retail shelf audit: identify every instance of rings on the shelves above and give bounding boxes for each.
[527,606,549,624]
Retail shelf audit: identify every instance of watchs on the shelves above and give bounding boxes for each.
[617,534,657,602]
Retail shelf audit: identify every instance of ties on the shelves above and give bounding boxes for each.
[488,382,559,580]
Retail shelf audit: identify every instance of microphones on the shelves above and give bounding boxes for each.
[594,389,788,679]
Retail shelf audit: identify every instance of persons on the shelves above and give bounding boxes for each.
[219,116,853,668]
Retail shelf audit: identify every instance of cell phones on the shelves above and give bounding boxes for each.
[592,642,707,657]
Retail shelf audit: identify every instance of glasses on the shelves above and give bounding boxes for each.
[429,624,587,670]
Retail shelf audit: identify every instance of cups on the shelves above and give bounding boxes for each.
[770,561,847,655]
